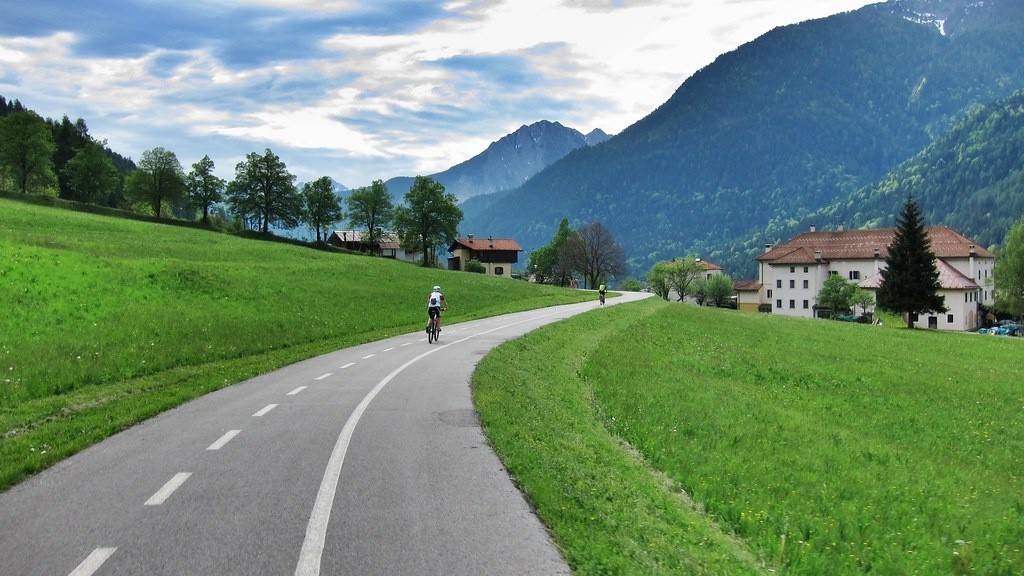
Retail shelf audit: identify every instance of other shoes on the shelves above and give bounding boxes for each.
[426,325,429,333]
[436,328,441,331]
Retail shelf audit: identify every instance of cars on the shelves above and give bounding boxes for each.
[979,320,1024,336]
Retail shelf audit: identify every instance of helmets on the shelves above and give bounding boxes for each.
[433,285,440,291]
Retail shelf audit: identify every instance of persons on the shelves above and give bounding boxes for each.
[425,286,448,333]
[599,282,607,303]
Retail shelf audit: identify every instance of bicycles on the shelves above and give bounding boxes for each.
[599,292,607,305]
[426,308,448,344]
